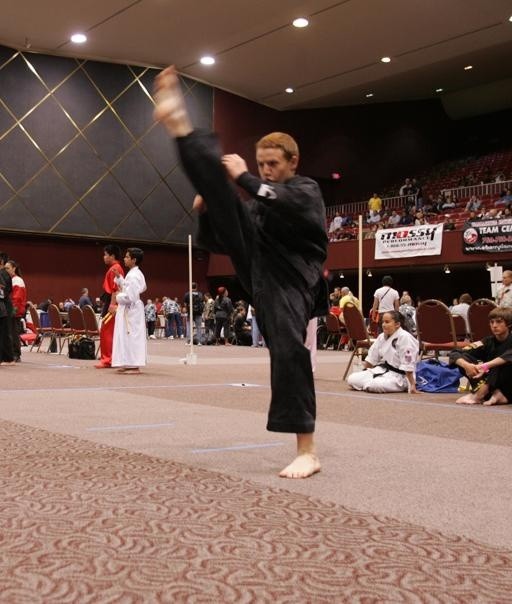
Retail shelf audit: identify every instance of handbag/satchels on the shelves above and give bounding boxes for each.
[66,336,98,361]
[410,356,461,395]
[190,331,254,347]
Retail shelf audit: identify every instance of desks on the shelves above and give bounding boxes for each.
[95,313,187,339]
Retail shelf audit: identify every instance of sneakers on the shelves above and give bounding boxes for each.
[147,334,185,340]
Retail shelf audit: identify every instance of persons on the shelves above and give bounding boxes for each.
[318,268,512,405]
[0,252,15,367]
[328,157,511,244]
[24,281,266,354]
[95,245,125,370]
[151,63,331,480]
[109,248,147,373]
[5,260,27,362]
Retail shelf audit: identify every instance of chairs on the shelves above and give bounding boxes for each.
[153,317,166,338]
[323,295,511,382]
[326,149,512,245]
[18,303,102,359]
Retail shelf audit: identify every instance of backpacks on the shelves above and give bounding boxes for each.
[186,293,205,314]
